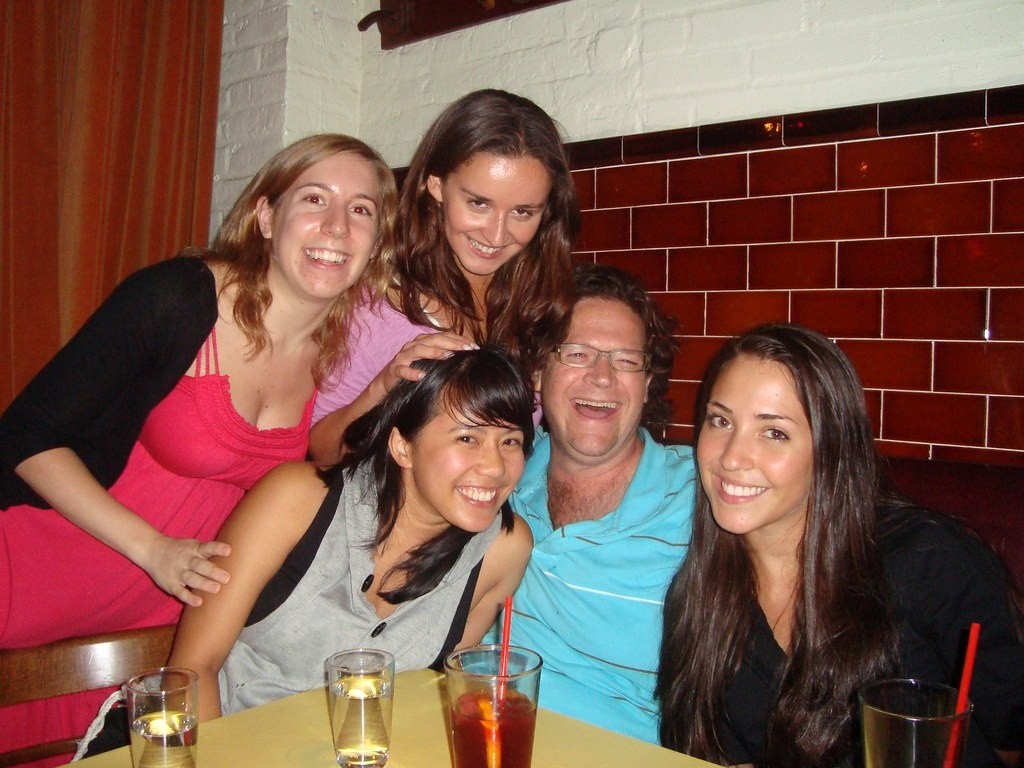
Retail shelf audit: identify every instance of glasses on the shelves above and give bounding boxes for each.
[549,342,652,371]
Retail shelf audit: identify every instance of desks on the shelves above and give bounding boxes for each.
[55,667,723,768]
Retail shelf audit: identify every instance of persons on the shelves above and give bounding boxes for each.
[308,86,580,474]
[0,131,399,768]
[68,348,535,760]
[653,323,1024,768]
[459,263,695,747]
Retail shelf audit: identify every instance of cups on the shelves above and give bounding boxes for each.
[326,647,396,768]
[857,679,975,768]
[125,667,199,768]
[443,643,544,768]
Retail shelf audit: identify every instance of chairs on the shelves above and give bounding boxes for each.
[0,624,179,768]
